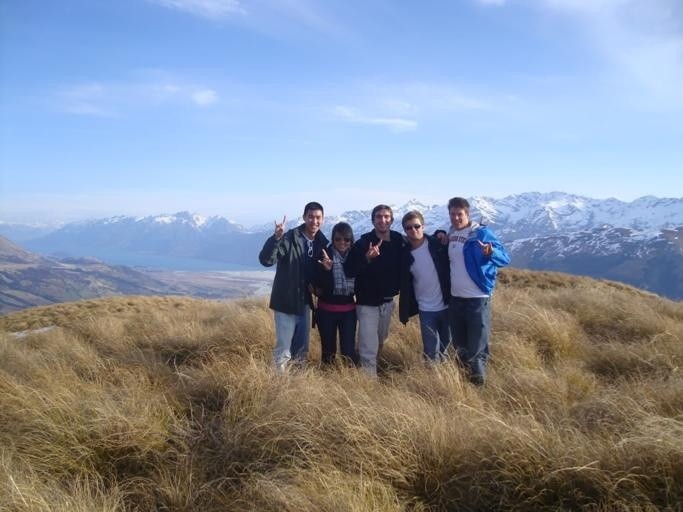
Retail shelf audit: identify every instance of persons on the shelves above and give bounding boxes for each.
[305,223,358,371]
[447,198,511,384]
[398,210,451,366]
[259,202,330,373]
[343,205,449,377]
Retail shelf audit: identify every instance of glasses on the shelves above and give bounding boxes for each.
[403,223,422,231]
[334,236,351,243]
